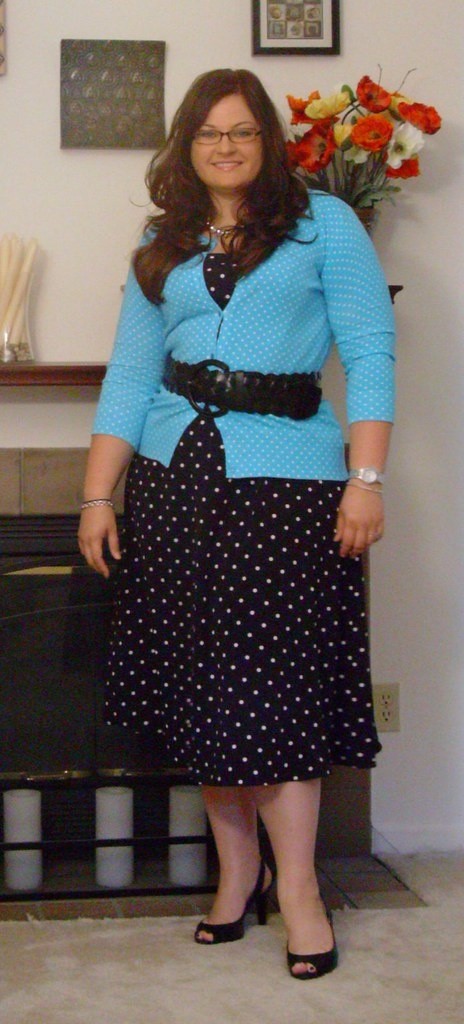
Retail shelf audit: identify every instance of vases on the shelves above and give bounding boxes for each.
[352,209,383,238]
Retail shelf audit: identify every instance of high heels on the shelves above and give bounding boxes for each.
[285,891,338,980]
[194,857,274,945]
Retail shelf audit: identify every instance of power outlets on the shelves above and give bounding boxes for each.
[373,681,399,733]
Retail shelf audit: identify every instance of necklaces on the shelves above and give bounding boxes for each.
[206,221,231,234]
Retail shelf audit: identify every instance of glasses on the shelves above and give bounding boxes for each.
[193,128,263,145]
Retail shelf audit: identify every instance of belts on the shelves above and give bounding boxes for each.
[162,350,323,420]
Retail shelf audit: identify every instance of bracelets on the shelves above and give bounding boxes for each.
[81,499,115,509]
[347,482,387,493]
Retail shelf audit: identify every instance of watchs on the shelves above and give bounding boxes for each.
[350,468,385,484]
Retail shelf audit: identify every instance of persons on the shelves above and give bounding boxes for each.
[78,68,398,981]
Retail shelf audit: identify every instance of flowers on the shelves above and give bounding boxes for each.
[284,65,442,205]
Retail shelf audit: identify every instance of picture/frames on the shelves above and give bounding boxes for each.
[250,0,340,56]
